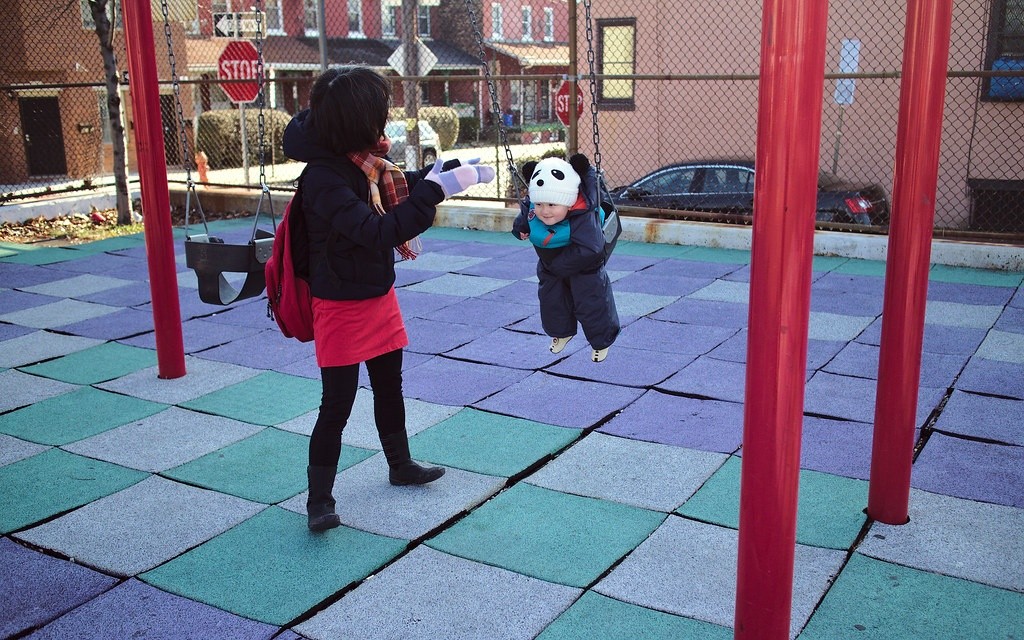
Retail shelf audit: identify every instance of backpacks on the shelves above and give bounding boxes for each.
[264,178,315,342]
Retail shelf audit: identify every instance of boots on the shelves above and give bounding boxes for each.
[306,459,340,531]
[379,428,445,486]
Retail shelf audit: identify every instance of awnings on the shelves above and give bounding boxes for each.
[184,37,485,71]
[486,40,570,67]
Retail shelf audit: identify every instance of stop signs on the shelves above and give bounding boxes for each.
[216,40,267,103]
[555,80,584,128]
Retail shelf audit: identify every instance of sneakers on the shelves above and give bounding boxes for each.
[549,335,573,354]
[591,347,610,362]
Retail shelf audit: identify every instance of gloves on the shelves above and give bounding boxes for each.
[461,158,495,184]
[424,158,479,200]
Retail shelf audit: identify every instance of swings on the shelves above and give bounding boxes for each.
[161,0,276,306]
[467,0,623,265]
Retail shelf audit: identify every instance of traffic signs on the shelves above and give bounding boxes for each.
[212,11,267,40]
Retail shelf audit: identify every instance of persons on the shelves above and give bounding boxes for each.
[511,153,621,362]
[283,66,495,529]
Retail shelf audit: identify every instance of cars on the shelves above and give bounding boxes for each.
[603,157,891,235]
[385,120,441,169]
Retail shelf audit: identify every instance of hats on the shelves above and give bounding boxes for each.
[522,153,590,207]
